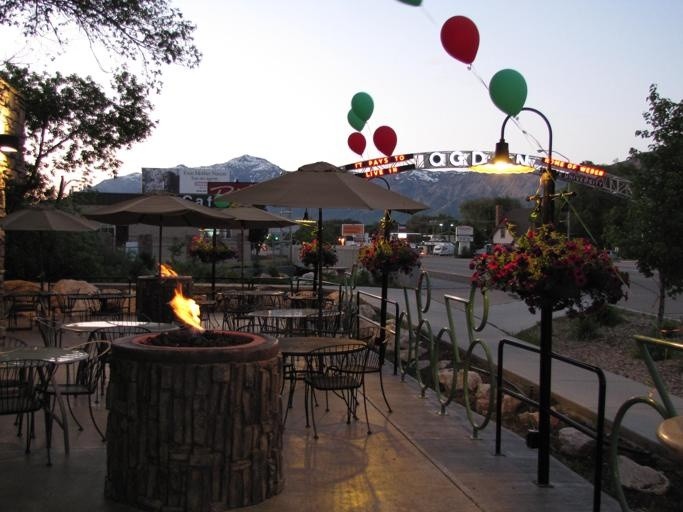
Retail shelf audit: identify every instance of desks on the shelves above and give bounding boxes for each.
[279,337,367,427]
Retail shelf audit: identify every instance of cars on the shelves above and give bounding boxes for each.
[431,244,450,256]
[471,243,491,258]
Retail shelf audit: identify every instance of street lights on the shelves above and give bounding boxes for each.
[294,206,323,294]
[438,222,444,234]
[533,146,572,245]
[449,222,454,235]
[469,107,561,488]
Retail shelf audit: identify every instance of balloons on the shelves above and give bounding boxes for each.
[489,69,528,118]
[347,92,398,158]
[439,16,481,65]
[398,0,424,7]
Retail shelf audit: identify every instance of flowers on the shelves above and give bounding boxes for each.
[466,212,632,320]
[186,232,236,264]
[298,239,338,269]
[356,226,423,281]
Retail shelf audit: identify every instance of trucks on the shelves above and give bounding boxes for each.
[416,245,427,256]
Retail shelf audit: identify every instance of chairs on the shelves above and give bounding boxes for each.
[324,326,393,425]
[180,278,382,351]
[2,278,181,467]
[304,342,371,438]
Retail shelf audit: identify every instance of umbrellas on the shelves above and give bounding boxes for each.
[81,194,237,277]
[212,161,430,334]
[214,207,305,317]
[0,208,101,319]
